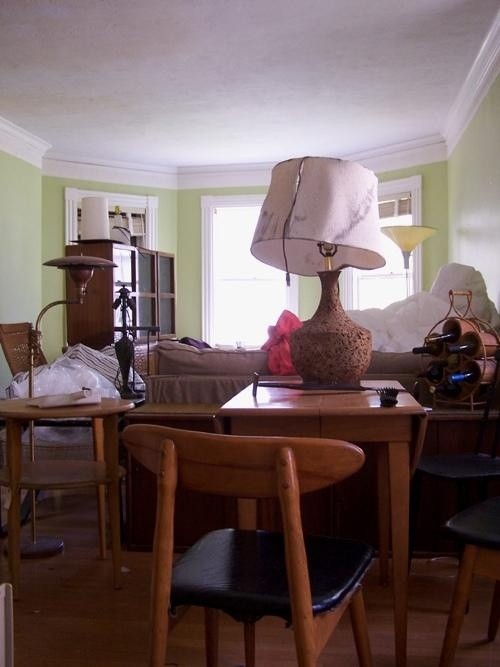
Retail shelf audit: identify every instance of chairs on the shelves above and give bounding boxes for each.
[408,381,499,666]
[118,422,378,665]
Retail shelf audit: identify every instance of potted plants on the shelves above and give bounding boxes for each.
[112,206,124,226]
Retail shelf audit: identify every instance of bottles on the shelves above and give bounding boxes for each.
[412,325,481,400]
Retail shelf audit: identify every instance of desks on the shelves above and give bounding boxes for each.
[1,397,134,601]
[219,379,425,665]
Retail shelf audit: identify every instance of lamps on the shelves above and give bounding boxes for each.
[378,226,437,299]
[250,159,387,387]
[1,256,118,560]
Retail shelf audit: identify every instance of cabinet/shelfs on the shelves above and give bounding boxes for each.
[66,243,175,350]
[126,403,498,554]
